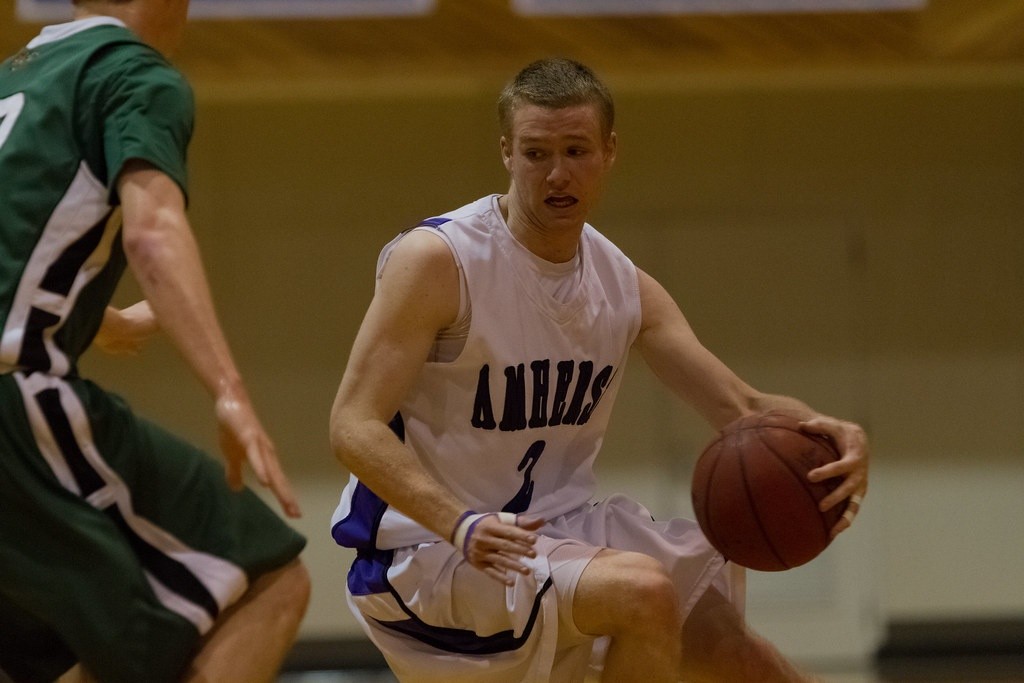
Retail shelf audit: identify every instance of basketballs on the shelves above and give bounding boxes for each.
[688,411,851,573]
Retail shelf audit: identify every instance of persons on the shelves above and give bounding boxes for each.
[0,0,312,683]
[327,55,869,683]
